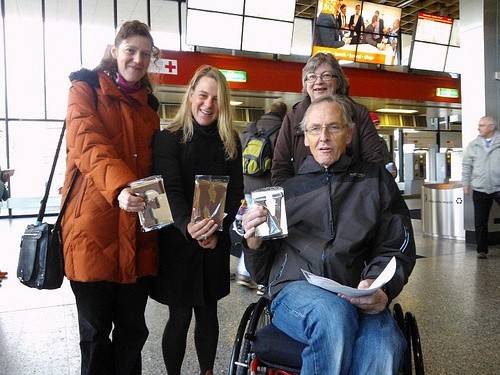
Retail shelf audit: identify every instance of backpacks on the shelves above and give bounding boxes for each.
[241,121,282,176]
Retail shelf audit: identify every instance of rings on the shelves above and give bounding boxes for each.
[203,234,208,238]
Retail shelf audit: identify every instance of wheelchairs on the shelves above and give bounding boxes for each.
[229,295,425,375]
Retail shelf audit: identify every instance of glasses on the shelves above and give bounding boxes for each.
[305,72,337,82]
[306,124,347,134]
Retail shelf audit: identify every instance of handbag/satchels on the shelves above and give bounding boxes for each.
[17,223,65,291]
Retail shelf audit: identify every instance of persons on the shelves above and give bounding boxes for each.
[242,94,416,375]
[369,113,397,178]
[461,115,500,258]
[316,0,399,65]
[58,20,160,375]
[151,65,244,375]
[271,52,397,189]
[0,167,9,202]
[236,101,287,295]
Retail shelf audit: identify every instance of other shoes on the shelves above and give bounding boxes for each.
[477,251,487,258]
